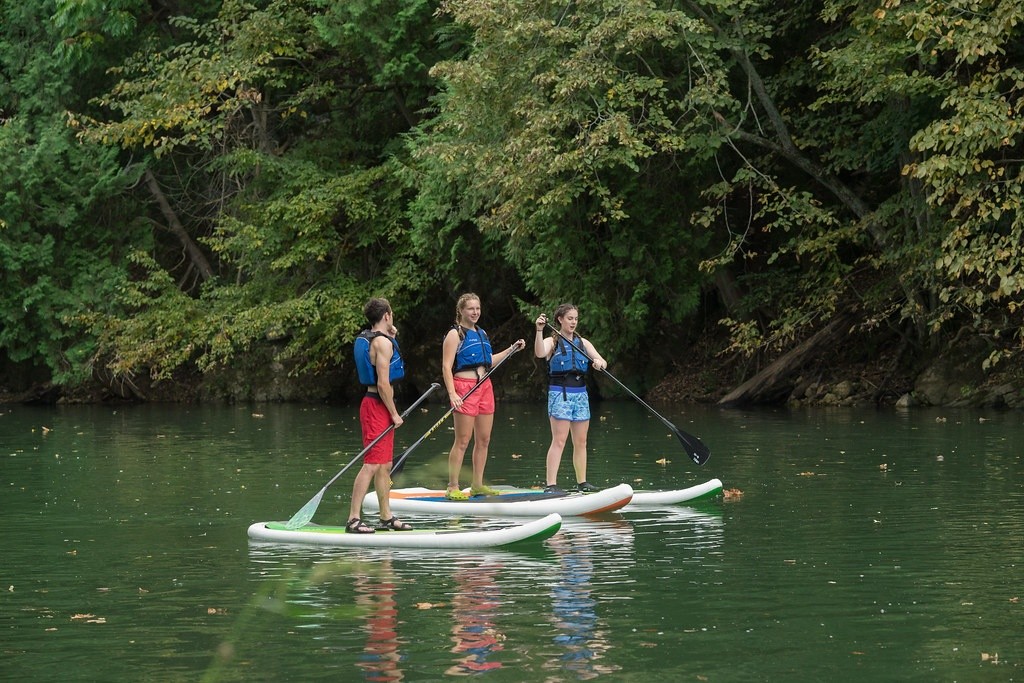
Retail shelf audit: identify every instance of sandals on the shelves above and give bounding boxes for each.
[345,518,375,533]
[379,516,413,531]
[445,487,469,501]
[470,484,500,496]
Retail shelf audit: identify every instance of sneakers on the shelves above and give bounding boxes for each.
[577,482,599,491]
[544,486,567,494]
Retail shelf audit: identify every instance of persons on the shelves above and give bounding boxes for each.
[344,299,413,534]
[442,293,526,500]
[534,303,607,496]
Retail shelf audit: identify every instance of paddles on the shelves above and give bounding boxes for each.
[388,341,523,483]
[284,380,441,530]
[542,317,712,468]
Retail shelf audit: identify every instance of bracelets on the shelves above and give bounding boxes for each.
[536,330,544,331]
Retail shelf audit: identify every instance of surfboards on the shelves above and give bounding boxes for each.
[249,512,564,550]
[462,478,722,507]
[359,482,633,519]
